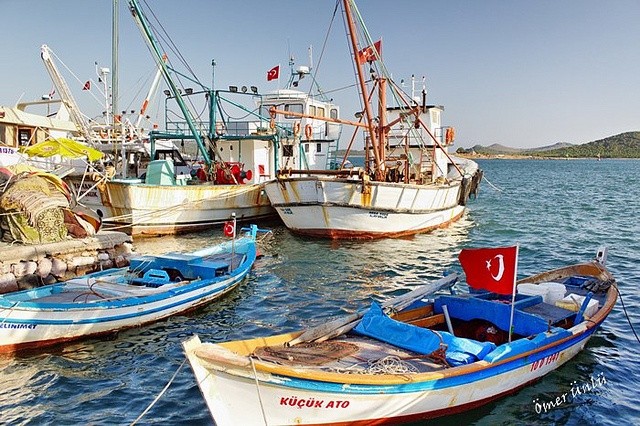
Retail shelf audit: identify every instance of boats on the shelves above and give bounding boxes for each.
[183,247,619,426]
[0,224,271,355]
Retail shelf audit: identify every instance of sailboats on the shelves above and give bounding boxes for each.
[0,45,202,230]
[263,0,482,240]
[0,53,168,176]
[106,1,353,238]
